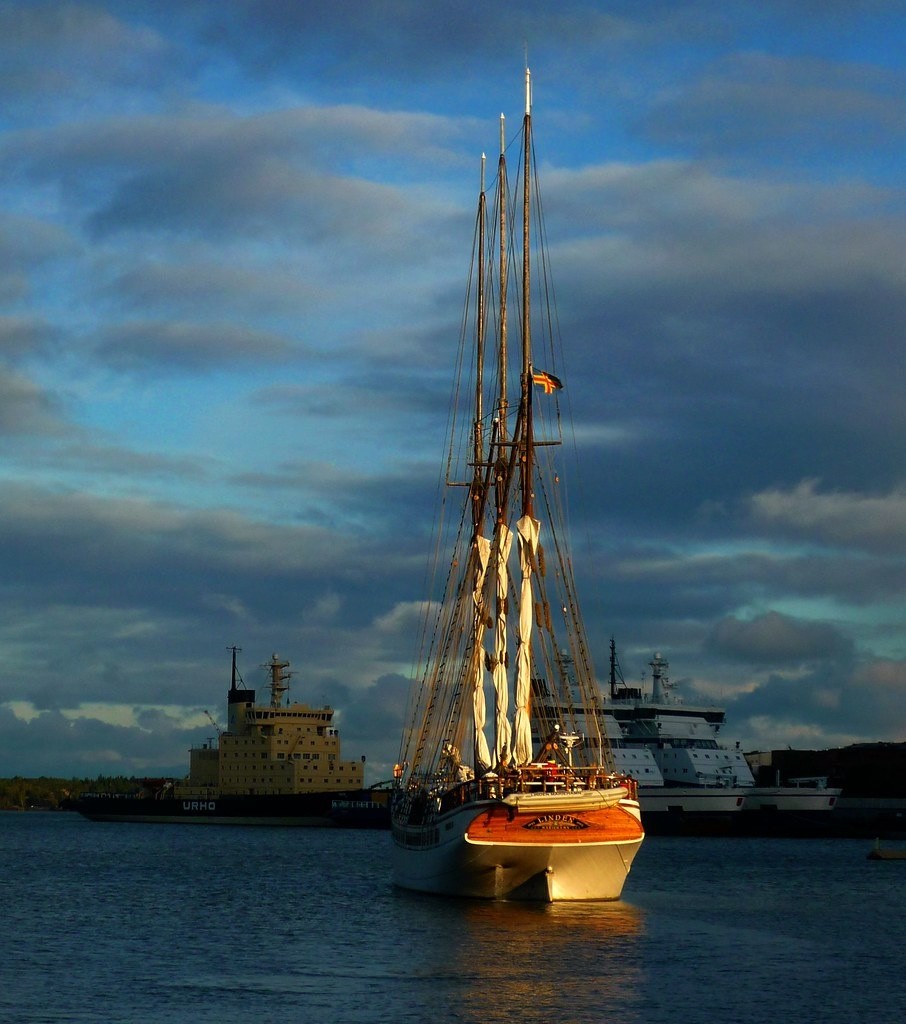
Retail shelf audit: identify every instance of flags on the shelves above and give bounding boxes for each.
[532,368,564,394]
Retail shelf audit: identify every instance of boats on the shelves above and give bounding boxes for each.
[61,643,407,830]
[413,632,845,841]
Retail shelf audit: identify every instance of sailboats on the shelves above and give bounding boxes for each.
[387,64,647,906]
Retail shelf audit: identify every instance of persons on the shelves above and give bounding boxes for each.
[508,763,521,793]
[499,774,505,799]
[278,728,283,734]
[482,767,498,799]
[542,756,558,792]
[625,774,638,784]
